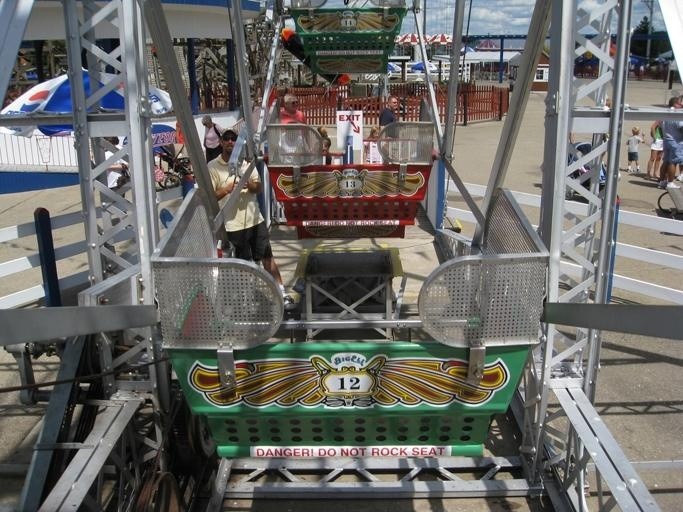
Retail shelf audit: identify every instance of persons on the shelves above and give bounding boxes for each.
[645,97,675,179]
[205,127,295,306]
[625,126,645,173]
[655,94,682,189]
[200,115,225,163]
[276,92,313,154]
[378,95,398,163]
[313,127,329,141]
[317,137,332,165]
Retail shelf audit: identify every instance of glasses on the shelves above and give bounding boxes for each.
[223,136,237,142]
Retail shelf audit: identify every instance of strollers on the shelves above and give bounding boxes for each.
[121,123,194,190]
[568,136,621,205]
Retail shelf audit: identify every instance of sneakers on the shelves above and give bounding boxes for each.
[627,168,683,189]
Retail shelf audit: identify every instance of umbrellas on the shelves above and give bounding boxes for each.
[410,62,438,73]
[580,48,673,75]
[386,62,402,74]
[0,65,174,138]
[392,32,453,60]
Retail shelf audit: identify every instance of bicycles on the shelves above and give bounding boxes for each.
[658,171,683,215]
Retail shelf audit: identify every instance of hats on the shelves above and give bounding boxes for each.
[220,127,239,136]
